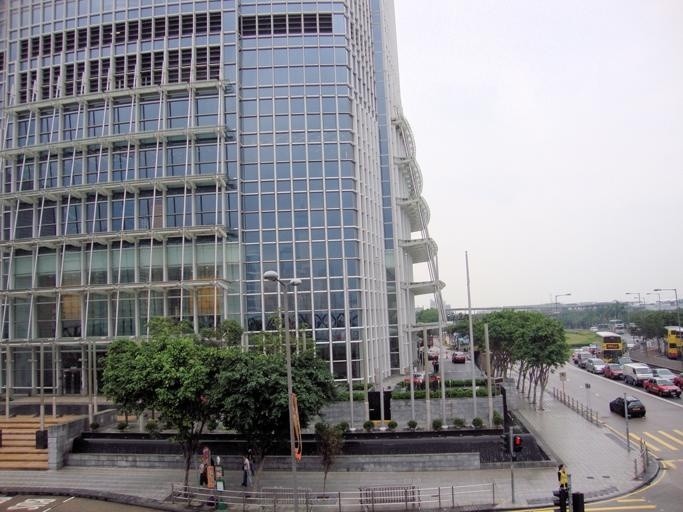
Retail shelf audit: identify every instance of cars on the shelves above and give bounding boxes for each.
[607,396,648,420]
[417,344,466,365]
[572,319,683,400]
[405,371,441,387]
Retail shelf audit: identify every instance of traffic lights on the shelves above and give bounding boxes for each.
[552,486,566,512]
[499,433,508,453]
[513,435,523,453]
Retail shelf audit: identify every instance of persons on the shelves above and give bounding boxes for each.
[433,357,438,372]
[557,463,569,497]
[431,358,435,369]
[196,445,257,488]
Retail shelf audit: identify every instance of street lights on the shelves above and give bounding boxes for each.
[624,288,682,338]
[554,291,573,321]
[261,269,305,511]
